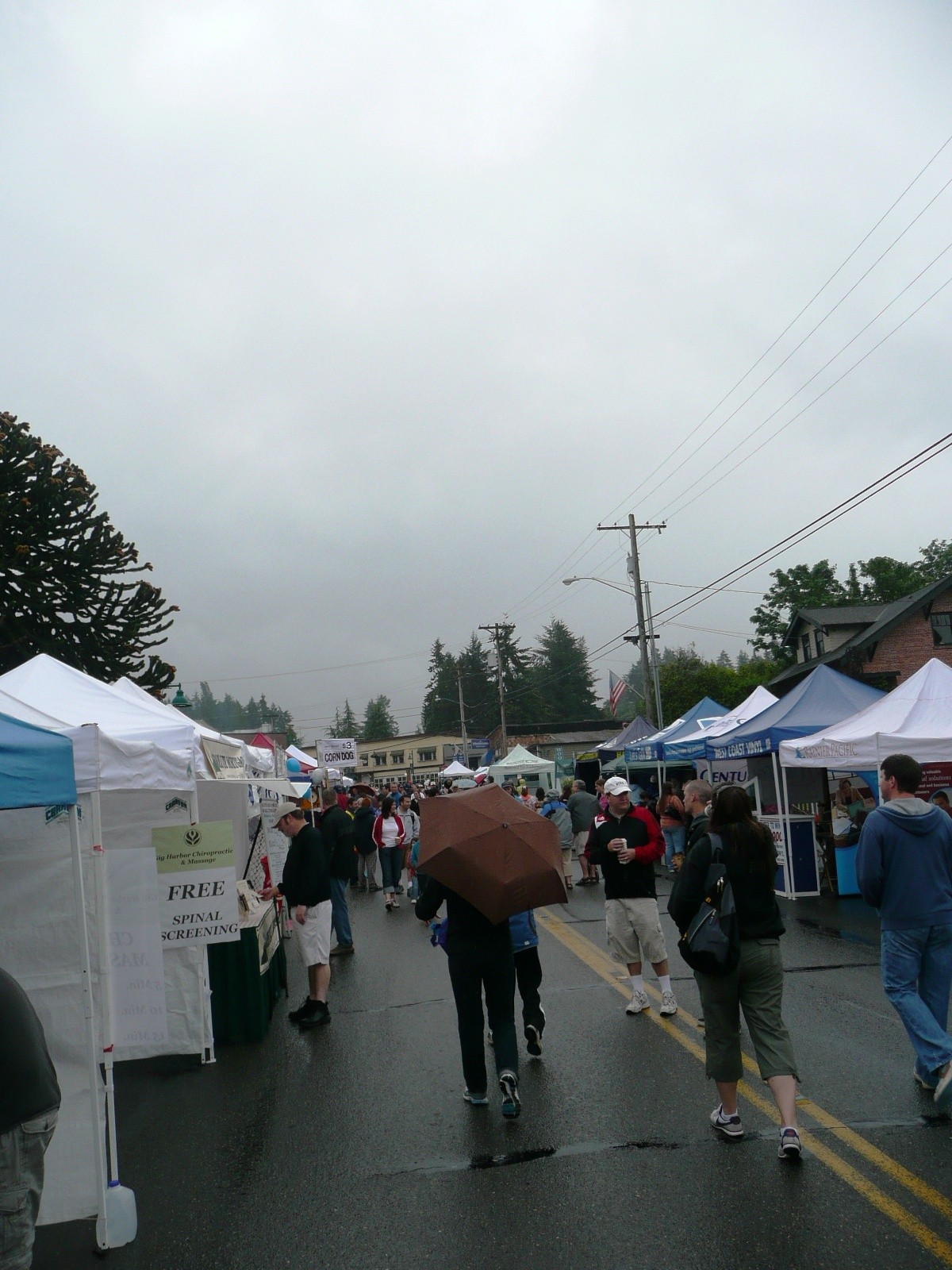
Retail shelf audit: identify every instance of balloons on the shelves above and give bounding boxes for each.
[286,758,301,773]
[303,788,310,799]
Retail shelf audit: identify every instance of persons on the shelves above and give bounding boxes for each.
[584,777,678,1015]
[298,779,866,1035]
[487,908,545,1056]
[415,879,522,1120]
[0,968,63,1270]
[932,791,952,819]
[258,803,333,1028]
[668,784,802,1160]
[855,754,952,1106]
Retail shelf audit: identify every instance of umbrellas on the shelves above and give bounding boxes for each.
[415,783,569,922]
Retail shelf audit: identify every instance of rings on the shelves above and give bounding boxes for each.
[625,857,628,860]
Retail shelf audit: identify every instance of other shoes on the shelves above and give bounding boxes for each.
[392,900,400,908]
[329,944,355,956]
[697,1017,705,1026]
[463,1086,488,1104]
[349,881,412,897]
[411,900,417,904]
[488,1031,493,1043]
[913,1066,935,1089]
[499,1073,521,1119]
[385,899,393,912]
[524,1025,542,1056]
[934,1059,952,1106]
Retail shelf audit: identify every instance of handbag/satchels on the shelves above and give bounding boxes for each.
[663,796,682,822]
[679,832,735,976]
[430,917,449,956]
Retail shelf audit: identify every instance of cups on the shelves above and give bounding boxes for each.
[618,838,627,863]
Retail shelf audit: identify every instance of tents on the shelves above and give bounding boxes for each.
[597,716,659,774]
[488,743,556,789]
[661,685,780,784]
[438,761,475,779]
[1,713,112,1252]
[705,665,889,899]
[624,696,730,796]
[778,658,952,901]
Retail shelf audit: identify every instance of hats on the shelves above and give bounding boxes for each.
[431,782,436,786]
[603,775,631,796]
[270,801,300,829]
[501,780,513,788]
[544,788,560,798]
[362,799,370,807]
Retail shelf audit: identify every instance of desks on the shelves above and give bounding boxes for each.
[206,900,289,1045]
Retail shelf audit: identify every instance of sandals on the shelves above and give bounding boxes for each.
[566,875,598,889]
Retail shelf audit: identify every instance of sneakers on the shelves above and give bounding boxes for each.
[625,989,650,1013]
[298,999,331,1031]
[777,1128,802,1158]
[659,990,678,1015]
[709,1103,743,1136]
[288,996,314,1021]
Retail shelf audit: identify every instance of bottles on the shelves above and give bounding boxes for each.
[95,1180,137,1250]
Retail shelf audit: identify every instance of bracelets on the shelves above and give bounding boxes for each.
[273,887,277,895]
